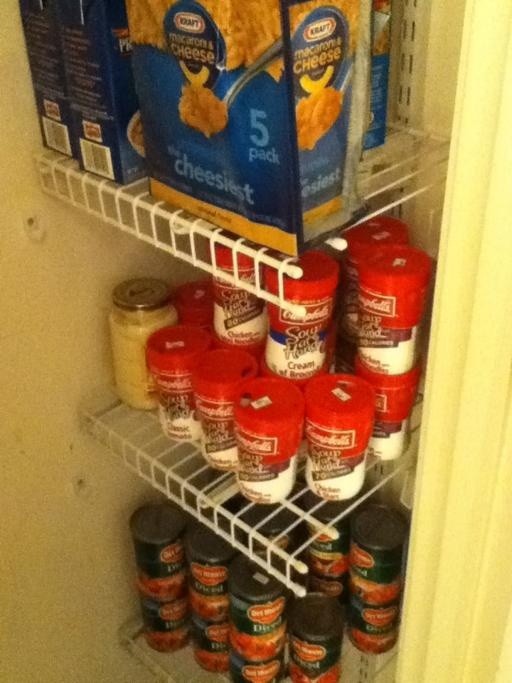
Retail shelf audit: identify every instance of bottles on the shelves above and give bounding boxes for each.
[105,214,433,506]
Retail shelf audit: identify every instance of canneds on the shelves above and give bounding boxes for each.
[130,478,409,683]
[108,276,178,411]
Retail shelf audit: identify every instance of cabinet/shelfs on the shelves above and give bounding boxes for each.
[36,0,451,683]
